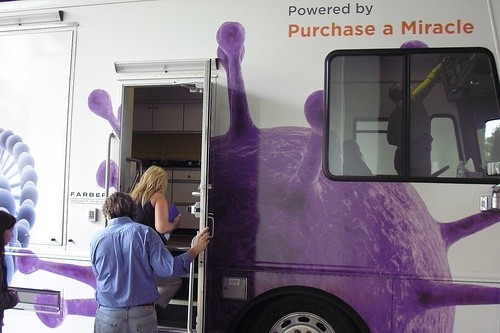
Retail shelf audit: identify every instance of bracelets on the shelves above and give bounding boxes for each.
[441,60,445,66]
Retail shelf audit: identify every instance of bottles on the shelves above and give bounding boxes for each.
[491,191,500,210]
[457,161,465,177]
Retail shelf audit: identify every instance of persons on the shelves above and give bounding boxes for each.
[386,55,451,176]
[126,165,182,326]
[90,192,212,333]
[0,210,20,333]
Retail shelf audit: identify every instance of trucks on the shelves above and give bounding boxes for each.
[0,0,500,332]
